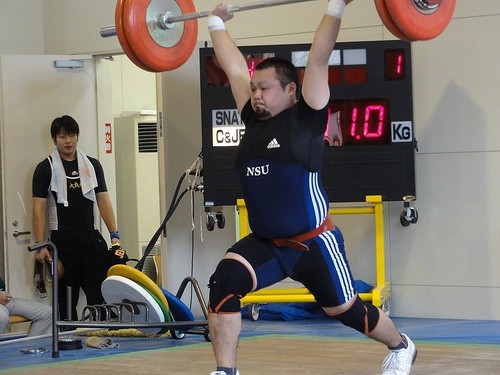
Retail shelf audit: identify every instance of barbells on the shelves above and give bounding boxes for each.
[99,0,455,72]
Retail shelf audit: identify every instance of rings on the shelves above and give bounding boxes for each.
[8,298,10,301]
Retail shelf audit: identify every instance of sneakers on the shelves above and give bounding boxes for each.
[209,369,240,375]
[381,333,419,375]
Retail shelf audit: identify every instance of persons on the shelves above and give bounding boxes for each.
[207,0,417,375]
[31,115,127,331]
[0,278,52,337]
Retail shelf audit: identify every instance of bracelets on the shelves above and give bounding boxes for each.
[110,231,119,239]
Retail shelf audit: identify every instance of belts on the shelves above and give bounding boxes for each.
[256,214,334,252]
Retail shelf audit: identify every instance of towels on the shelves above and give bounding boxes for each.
[50,147,99,207]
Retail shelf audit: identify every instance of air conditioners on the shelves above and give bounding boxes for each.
[116,116,162,290]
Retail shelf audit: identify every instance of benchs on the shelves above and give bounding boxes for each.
[9,315,31,336]
